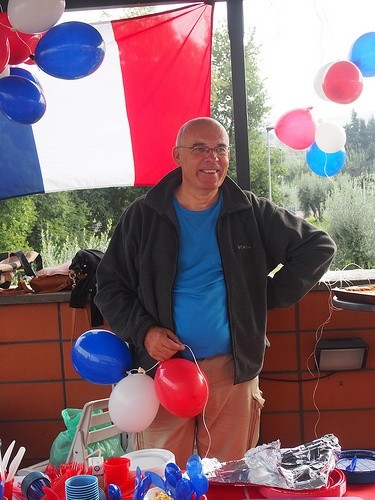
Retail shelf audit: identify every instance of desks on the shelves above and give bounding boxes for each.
[332,283,375,312]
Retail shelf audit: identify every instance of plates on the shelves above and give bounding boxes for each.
[119,449,178,500]
[258,449,375,500]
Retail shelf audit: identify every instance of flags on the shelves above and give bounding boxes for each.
[0,2,214,200]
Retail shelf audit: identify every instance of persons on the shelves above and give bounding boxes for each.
[95,117,338,469]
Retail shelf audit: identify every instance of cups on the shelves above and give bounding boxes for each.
[0,458,136,500]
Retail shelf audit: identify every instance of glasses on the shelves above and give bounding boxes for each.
[179,145,230,155]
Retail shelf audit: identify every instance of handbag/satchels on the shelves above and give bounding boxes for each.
[29,260,76,293]
[0,250,44,289]
[69,249,104,327]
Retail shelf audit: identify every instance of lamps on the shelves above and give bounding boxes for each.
[315,337,368,372]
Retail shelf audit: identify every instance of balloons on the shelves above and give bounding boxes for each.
[274,32,374,177]
[0,0,106,124]
[71,329,209,433]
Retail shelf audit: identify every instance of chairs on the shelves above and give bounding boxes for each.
[16,397,128,476]
